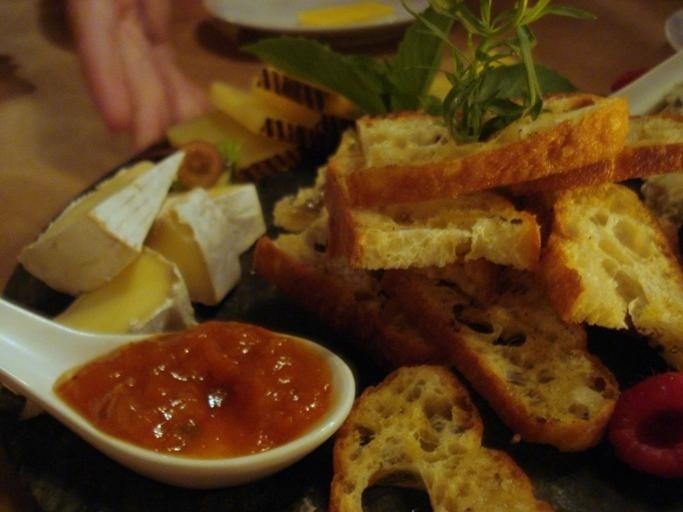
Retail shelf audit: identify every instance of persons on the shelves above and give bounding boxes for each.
[73,1,209,147]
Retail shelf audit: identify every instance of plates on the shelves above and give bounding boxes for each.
[207,2,428,33]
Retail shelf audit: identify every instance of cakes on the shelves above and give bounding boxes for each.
[19,151,269,418]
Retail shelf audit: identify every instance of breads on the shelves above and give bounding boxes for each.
[250,78,683,512]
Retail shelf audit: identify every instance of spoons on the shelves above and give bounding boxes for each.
[0,299,356,485]
